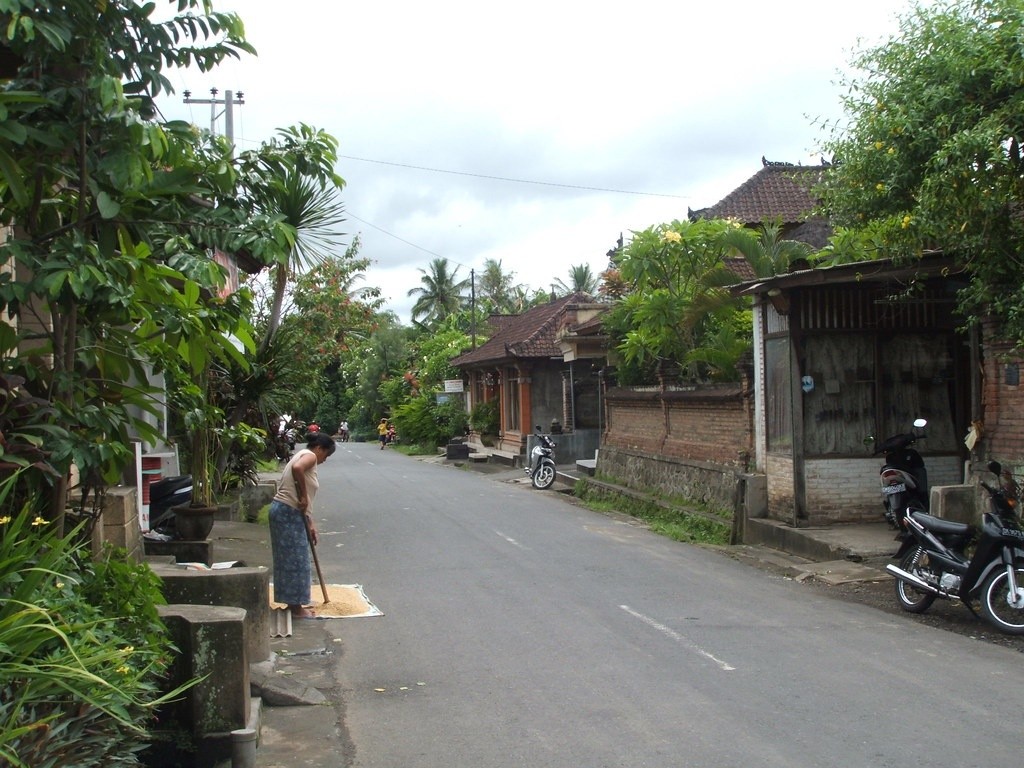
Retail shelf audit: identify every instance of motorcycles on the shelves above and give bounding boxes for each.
[275,436,293,463]
[142,475,211,534]
[307,426,320,433]
[884,459,1023,636]
[523,425,557,489]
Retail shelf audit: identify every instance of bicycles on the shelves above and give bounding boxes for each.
[341,430,348,442]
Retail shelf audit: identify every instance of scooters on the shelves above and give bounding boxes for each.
[863,419,931,532]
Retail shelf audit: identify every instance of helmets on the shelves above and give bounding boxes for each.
[312,421,315,424]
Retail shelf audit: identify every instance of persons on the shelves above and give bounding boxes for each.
[377,418,388,450]
[308,420,320,433]
[385,424,396,443]
[340,419,349,442]
[268,431,337,619]
[277,409,294,438]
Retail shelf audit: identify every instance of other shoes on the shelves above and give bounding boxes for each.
[381,445,384,449]
